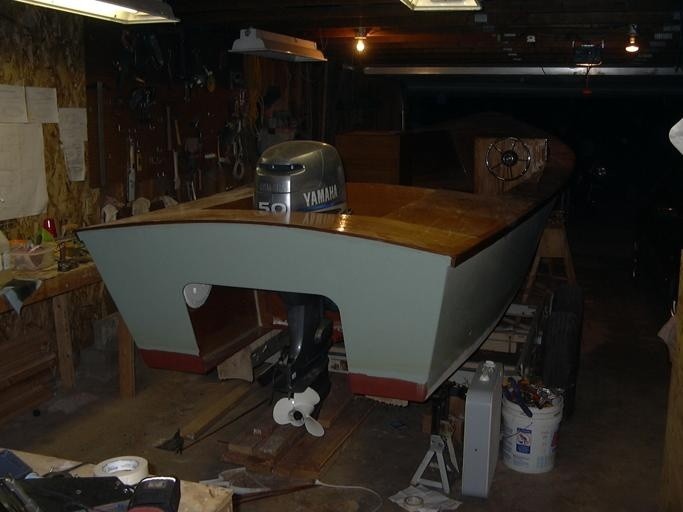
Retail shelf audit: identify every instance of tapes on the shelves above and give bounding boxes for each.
[93,456,149,486]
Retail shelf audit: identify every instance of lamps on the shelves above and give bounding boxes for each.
[353,16,367,40]
[229,26,332,67]
[16,0,181,26]
[568,24,642,69]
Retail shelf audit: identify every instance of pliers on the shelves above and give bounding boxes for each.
[503,377,532,417]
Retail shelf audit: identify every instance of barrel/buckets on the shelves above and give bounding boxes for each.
[500,394,565,476]
